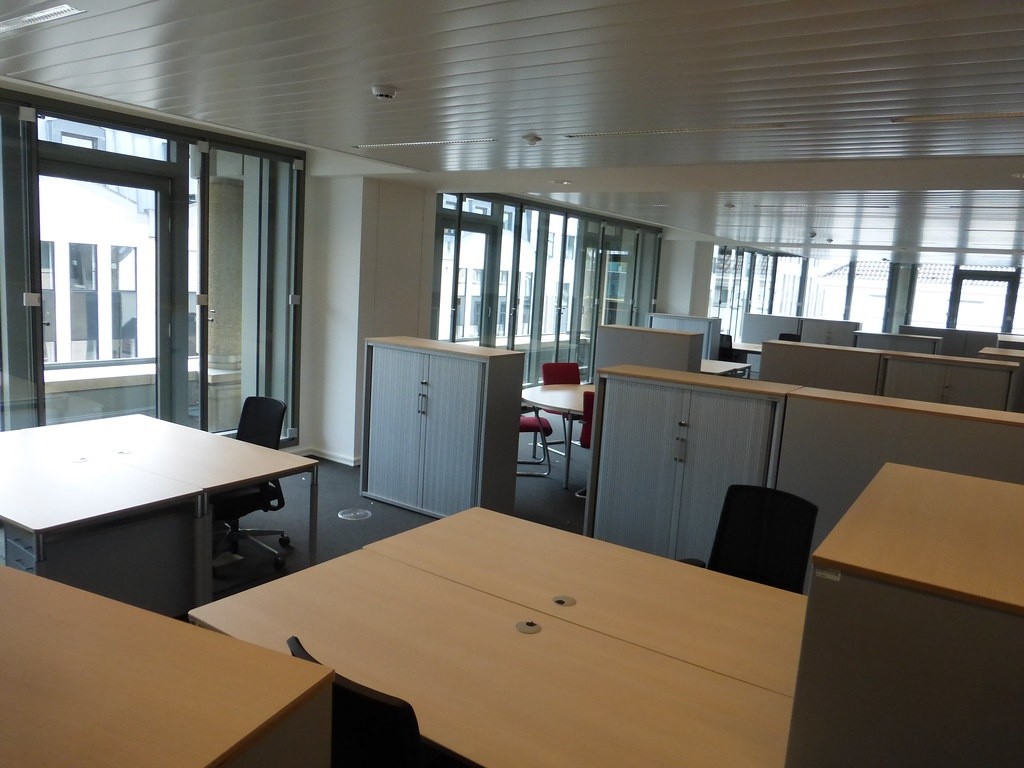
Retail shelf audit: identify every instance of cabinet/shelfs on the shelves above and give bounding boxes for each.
[360,336,523,516]
[585,313,1024,768]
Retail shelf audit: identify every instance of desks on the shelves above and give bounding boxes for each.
[700,359,752,378]
[522,383,595,489]
[0,413,319,622]
[732,341,762,365]
[0,528,331,768]
[187,506,808,768]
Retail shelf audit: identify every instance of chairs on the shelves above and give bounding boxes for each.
[718,334,732,362]
[571,391,595,499]
[779,334,802,342]
[709,483,819,591]
[541,362,582,458]
[212,396,290,567]
[287,637,424,768]
[516,407,552,478]
